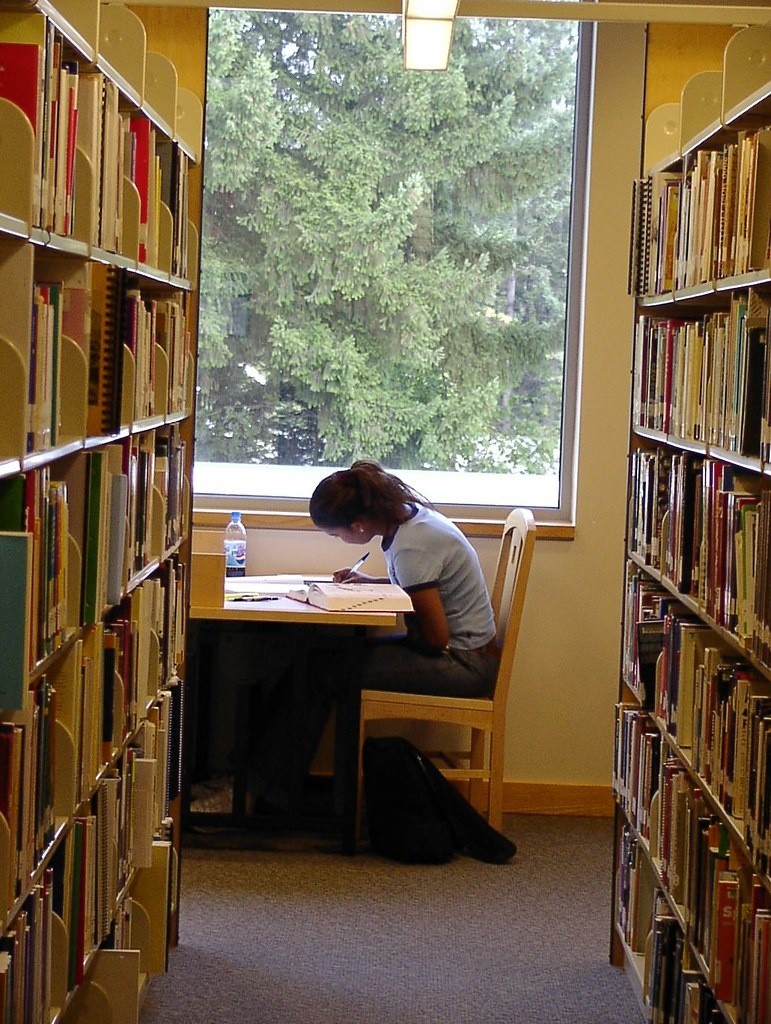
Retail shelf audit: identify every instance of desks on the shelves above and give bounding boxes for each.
[179,551,398,842]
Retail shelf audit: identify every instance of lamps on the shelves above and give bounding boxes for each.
[401,0,462,71]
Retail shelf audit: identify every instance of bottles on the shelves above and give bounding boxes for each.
[224,511,246,576]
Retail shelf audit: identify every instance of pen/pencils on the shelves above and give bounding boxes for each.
[339,552,370,583]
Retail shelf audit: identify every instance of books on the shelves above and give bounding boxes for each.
[286,580,417,615]
[611,124,771,1024]
[0,5,195,1023]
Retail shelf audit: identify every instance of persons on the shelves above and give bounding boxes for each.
[186,460,499,834]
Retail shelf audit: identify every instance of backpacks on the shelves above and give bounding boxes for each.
[361,736,517,866]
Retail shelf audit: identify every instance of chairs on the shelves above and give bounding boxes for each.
[350,506,537,852]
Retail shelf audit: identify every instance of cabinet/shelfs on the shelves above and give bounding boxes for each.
[0,1,210,1024]
[608,24,771,1023]
